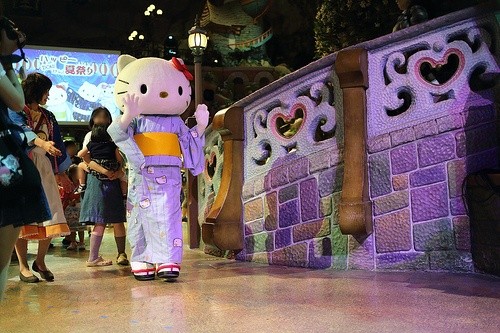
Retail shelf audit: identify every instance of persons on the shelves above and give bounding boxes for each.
[0,15,74,283]
[73,106,129,266]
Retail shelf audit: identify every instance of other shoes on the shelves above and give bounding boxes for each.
[67,243,77,249]
[19,272,39,283]
[74,185,86,194]
[79,244,85,249]
[32,260,54,280]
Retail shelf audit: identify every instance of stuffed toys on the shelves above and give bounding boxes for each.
[107,54,209,282]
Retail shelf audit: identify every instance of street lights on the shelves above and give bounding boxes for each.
[188,14,209,116]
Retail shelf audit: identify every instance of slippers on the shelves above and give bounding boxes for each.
[86,256,112,267]
[116,256,129,264]
